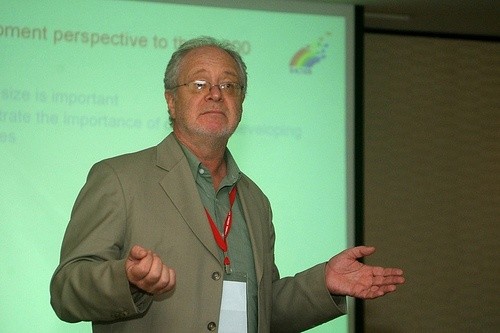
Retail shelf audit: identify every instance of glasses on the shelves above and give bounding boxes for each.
[168,80,243,98]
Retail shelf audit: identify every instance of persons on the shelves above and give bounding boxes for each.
[48,37,405,333]
